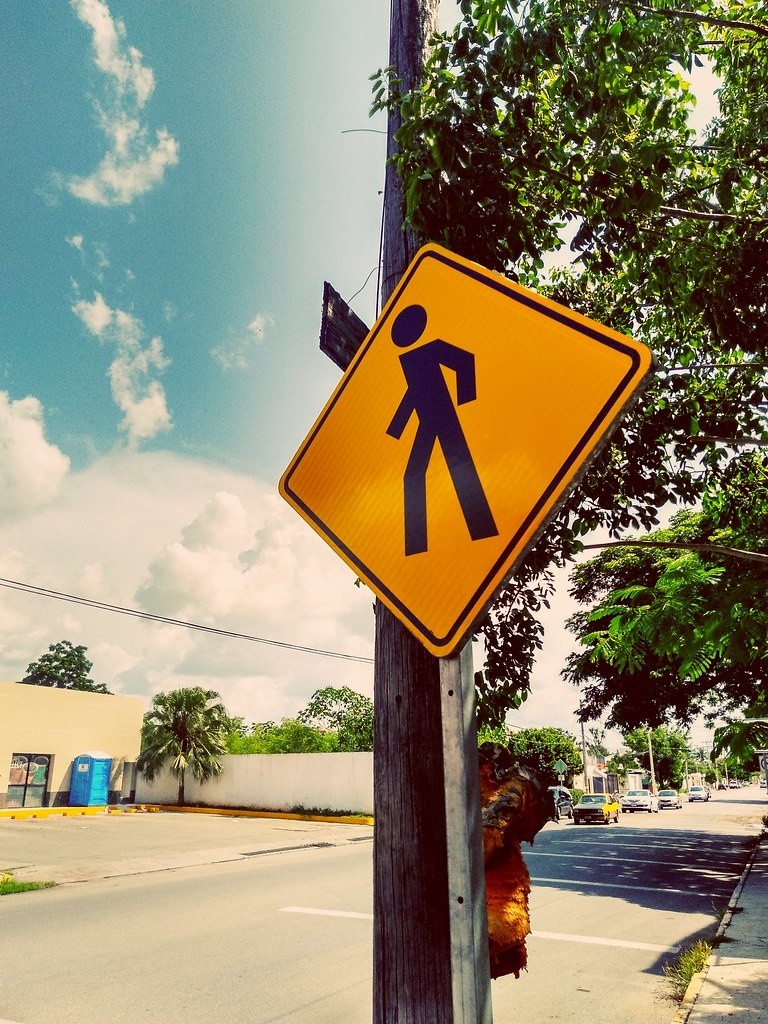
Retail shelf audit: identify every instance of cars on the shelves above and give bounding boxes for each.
[742,780,749,787]
[571,793,620,824]
[759,781,767,788]
[657,790,682,809]
[729,781,740,789]
[547,786,574,819]
[621,790,659,813]
[718,784,726,790]
[687,786,708,801]
